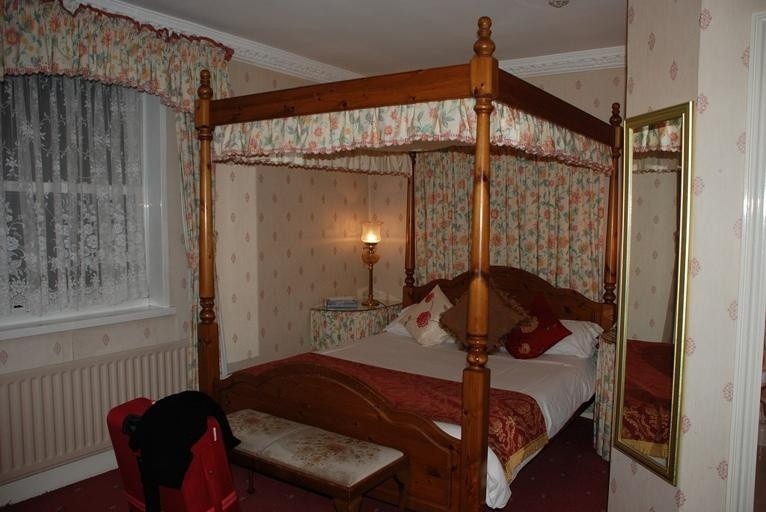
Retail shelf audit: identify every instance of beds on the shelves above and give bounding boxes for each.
[619,339,673,471]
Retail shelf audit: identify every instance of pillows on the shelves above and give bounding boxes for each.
[503,292,571,358]
[400,286,453,347]
[385,303,454,343]
[541,319,604,357]
[441,281,527,354]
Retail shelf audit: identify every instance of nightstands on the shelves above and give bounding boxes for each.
[309,298,401,350]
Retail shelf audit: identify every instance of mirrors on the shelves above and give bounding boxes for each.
[611,99,695,487]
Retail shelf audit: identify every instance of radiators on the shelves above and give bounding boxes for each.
[0,339,190,485]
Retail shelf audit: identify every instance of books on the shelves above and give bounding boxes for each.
[324,295,361,308]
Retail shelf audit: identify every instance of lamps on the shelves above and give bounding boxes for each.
[360,222,381,305]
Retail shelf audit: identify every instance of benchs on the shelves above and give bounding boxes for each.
[226,409,411,510]
